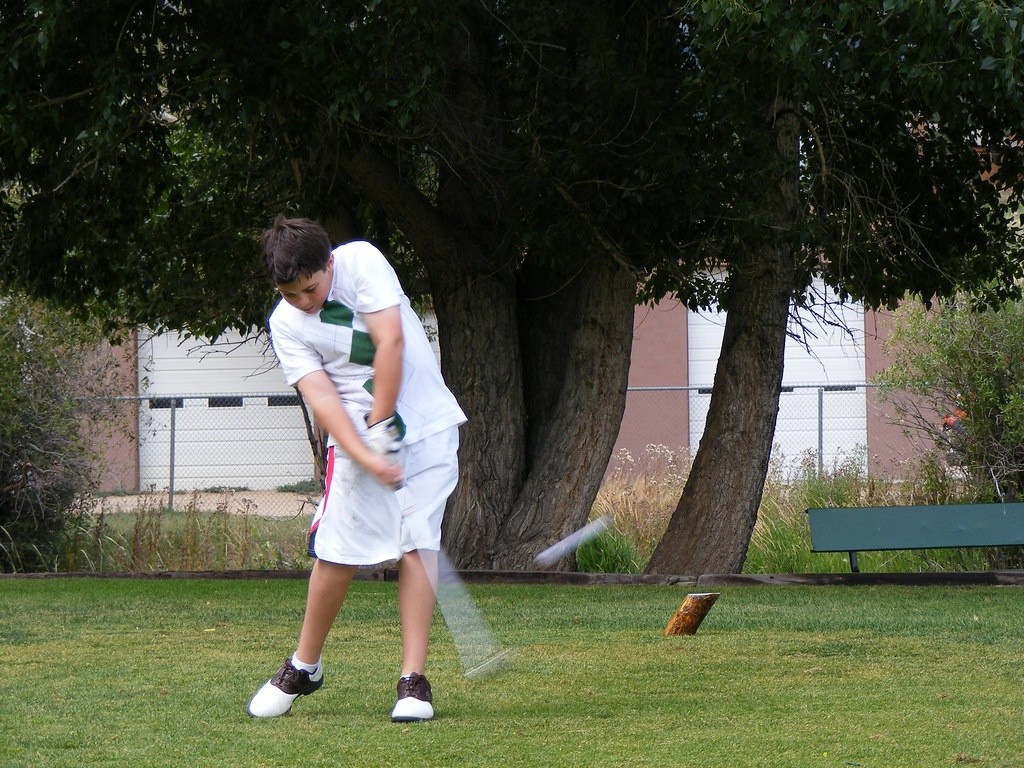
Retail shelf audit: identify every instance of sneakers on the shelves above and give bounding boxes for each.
[247,658,324,719]
[391,672,435,720]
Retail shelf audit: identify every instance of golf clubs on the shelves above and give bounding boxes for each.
[363,412,522,682]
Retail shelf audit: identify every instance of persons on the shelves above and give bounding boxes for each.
[249,218,468,722]
